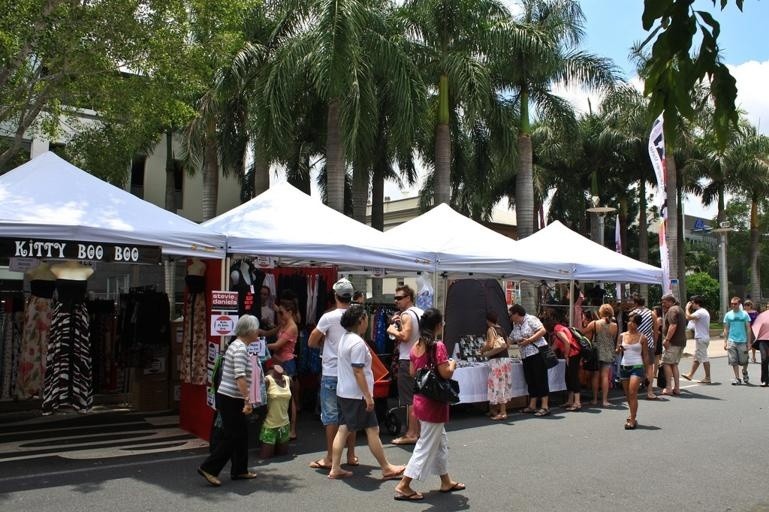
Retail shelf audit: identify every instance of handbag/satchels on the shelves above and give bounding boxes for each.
[411,341,460,405]
[481,327,507,358]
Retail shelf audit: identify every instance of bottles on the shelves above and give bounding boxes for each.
[389,323,398,340]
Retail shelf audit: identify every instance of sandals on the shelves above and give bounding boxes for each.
[565,402,582,411]
[517,406,537,414]
[231,471,257,481]
[489,414,508,420]
[197,466,222,486]
[533,408,551,417]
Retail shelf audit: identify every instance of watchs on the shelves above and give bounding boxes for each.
[664,337,672,342]
[242,395,253,404]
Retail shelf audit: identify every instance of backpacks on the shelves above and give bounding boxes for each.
[556,326,591,356]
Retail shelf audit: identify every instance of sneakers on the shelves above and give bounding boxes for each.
[742,369,749,384]
[731,378,741,385]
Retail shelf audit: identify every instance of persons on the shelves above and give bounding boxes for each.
[651,304,662,380]
[508,302,550,417]
[307,276,360,470]
[627,294,665,402]
[479,310,512,421]
[614,311,650,431]
[386,285,426,447]
[656,291,686,396]
[757,340,769,388]
[681,295,713,384]
[743,300,762,364]
[392,307,467,502]
[265,298,302,441]
[259,283,278,333]
[354,288,365,304]
[540,315,592,411]
[722,296,752,385]
[194,312,259,487]
[581,303,618,409]
[326,302,407,481]
[262,363,298,460]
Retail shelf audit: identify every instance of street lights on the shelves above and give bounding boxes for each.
[704,216,739,327]
[585,195,620,250]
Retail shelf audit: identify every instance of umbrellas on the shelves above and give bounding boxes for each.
[750,309,769,342]
[385,199,578,283]
[0,150,228,261]
[517,218,665,289]
[200,175,441,274]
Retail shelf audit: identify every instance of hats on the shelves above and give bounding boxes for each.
[332,278,355,298]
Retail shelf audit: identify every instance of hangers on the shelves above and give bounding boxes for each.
[131,284,160,293]
[365,302,398,314]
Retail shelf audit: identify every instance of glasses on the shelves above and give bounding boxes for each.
[279,310,289,316]
[731,303,738,305]
[394,295,408,301]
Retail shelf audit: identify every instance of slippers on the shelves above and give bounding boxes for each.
[308,457,332,468]
[439,482,466,493]
[346,456,360,466]
[327,470,353,479]
[389,434,418,445]
[626,415,632,421]
[381,464,407,480]
[625,420,637,429]
[698,379,711,384]
[682,374,691,381]
[659,389,673,395]
[647,395,659,400]
[672,388,681,395]
[393,490,425,500]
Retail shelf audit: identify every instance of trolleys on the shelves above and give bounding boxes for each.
[372,379,401,436]
